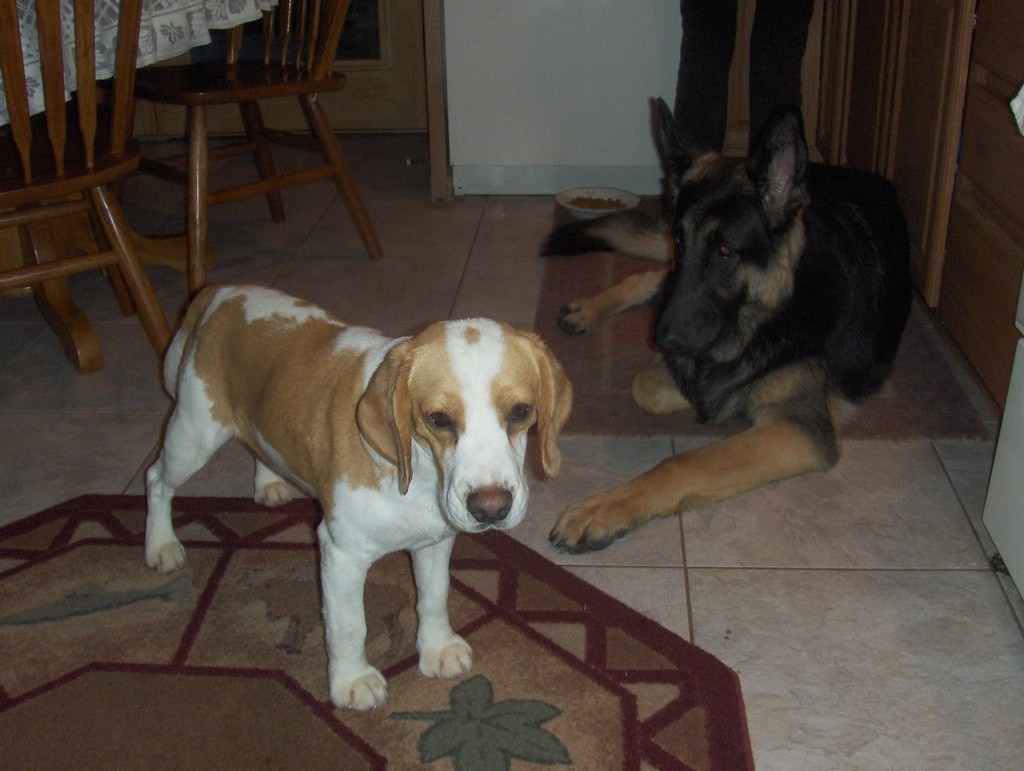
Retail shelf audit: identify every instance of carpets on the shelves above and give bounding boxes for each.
[524,195,988,442]
[0,492,756,771]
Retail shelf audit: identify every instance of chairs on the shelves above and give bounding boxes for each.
[98,0,384,304]
[0,0,216,377]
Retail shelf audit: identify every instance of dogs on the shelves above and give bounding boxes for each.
[144,283,575,712]
[538,97,914,556]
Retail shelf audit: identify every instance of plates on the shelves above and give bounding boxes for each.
[554,186,642,221]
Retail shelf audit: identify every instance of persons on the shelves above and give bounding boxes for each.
[676,1,815,159]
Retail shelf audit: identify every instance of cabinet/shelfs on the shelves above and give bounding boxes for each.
[979,270,1024,602]
